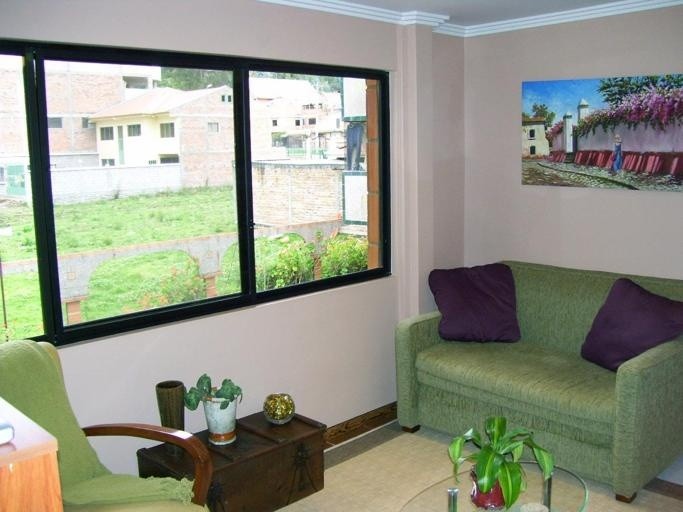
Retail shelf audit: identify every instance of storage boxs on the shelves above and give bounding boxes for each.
[134,409,327,511]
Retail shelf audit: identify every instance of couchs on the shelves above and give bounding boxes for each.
[391,259,682,504]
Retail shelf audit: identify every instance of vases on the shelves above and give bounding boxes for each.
[152,378,187,458]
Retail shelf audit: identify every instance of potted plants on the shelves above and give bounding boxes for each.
[182,374,242,446]
[446,412,559,508]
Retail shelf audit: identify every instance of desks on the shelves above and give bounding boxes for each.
[403,461,587,510]
[1,388,62,511]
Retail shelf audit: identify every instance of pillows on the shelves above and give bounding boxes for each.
[428,262,522,344]
[581,278,681,372]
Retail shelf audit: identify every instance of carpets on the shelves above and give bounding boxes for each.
[275,414,683,511]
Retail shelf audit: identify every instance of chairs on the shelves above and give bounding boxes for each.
[1,341,212,511]
